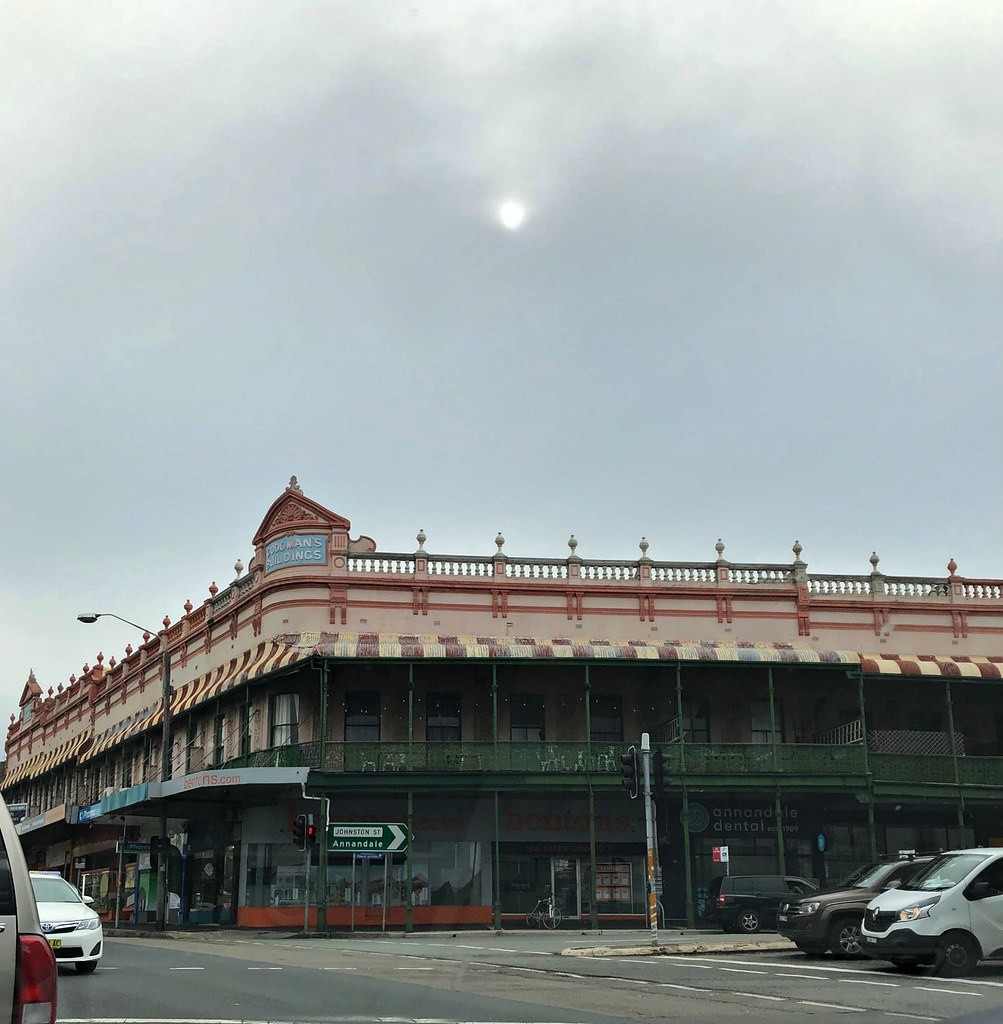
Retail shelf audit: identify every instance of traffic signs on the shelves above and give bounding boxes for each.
[326,822,416,853]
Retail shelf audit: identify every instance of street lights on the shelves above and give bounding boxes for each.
[76,611,170,932]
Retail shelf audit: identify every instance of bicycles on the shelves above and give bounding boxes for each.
[524,892,562,930]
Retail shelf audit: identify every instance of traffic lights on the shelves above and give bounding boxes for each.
[292,814,307,851]
[620,745,638,800]
[652,749,674,794]
[306,825,316,845]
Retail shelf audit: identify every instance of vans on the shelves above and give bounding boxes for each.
[704,875,820,934]
[0,790,58,1023]
[859,847,1003,979]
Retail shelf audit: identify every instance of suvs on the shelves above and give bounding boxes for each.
[776,847,945,961]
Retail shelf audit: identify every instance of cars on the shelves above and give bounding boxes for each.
[28,870,104,974]
